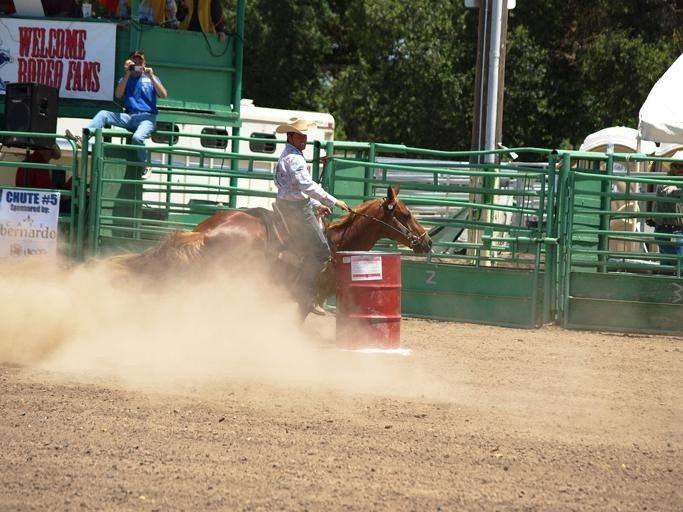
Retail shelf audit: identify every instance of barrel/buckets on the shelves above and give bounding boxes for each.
[334,251,401,350]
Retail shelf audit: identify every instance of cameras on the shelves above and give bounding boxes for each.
[129,64,144,72]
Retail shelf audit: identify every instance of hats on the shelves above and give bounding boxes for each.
[275,115,318,136]
[129,50,145,61]
[28,138,61,160]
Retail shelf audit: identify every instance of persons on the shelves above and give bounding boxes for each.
[14,143,61,190]
[268,113,352,317]
[64,50,169,181]
[648,151,683,276]
[137,1,181,30]
[174,1,227,44]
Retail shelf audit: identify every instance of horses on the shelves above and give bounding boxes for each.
[108,183,433,324]
[14,143,89,244]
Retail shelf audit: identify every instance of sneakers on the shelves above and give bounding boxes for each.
[64,129,83,149]
[291,290,327,313]
[139,165,152,180]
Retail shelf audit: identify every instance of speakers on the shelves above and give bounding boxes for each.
[1,83,59,149]
